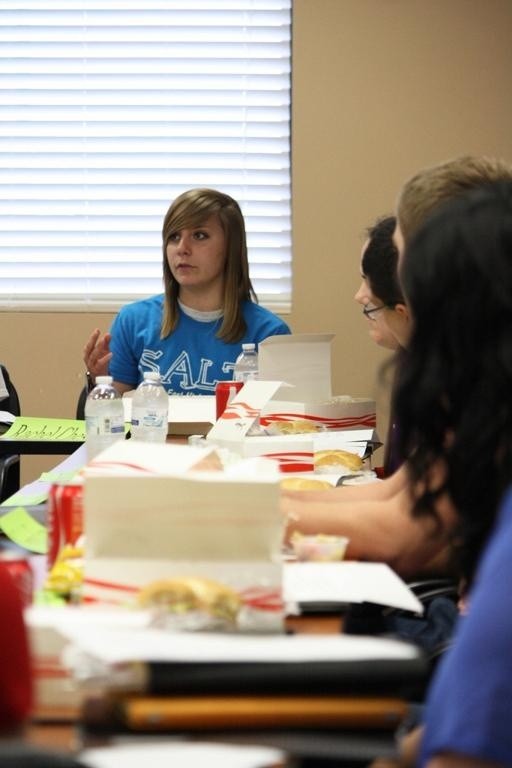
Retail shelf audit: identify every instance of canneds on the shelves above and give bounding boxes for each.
[215,380,244,419]
[46,479,84,573]
[0,550,34,732]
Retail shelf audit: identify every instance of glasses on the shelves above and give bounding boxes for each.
[362,301,405,321]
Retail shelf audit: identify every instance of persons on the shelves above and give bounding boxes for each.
[83,188,292,395]
[280,157,512,768]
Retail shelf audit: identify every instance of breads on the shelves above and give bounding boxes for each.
[265,418,319,433]
[278,477,330,490]
[312,449,362,473]
[135,576,239,618]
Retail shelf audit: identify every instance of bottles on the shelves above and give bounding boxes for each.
[234,343,260,382]
[84,371,171,464]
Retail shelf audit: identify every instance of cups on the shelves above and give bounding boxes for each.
[292,533,350,561]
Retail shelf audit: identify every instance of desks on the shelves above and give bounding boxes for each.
[0,424,388,761]
